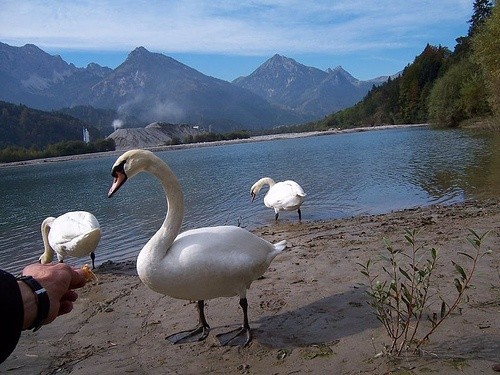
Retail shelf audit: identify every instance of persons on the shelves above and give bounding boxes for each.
[0,262,88,367]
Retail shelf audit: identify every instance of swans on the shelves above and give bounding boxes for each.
[250,176,308,222]
[106,149,289,351]
[39,210,102,272]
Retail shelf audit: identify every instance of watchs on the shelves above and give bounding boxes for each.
[15,276,49,333]
